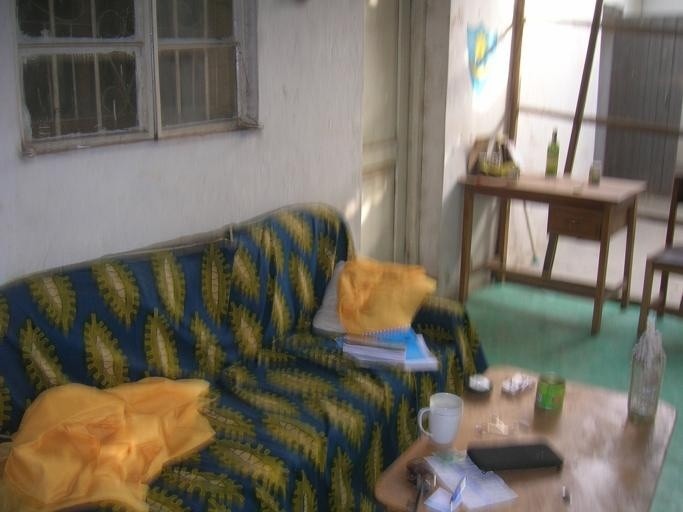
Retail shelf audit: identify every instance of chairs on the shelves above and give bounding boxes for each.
[637,175,683,338]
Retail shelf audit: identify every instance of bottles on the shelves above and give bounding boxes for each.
[544,129,559,179]
[627,311,666,426]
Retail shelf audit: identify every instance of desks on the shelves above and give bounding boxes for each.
[456,173,646,336]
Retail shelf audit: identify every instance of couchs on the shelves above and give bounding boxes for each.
[0,203,488,512]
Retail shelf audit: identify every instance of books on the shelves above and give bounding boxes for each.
[330,325,439,374]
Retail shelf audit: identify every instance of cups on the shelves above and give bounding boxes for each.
[416,392,464,445]
[588,161,599,185]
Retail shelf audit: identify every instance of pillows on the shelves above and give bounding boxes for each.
[311,259,348,335]
